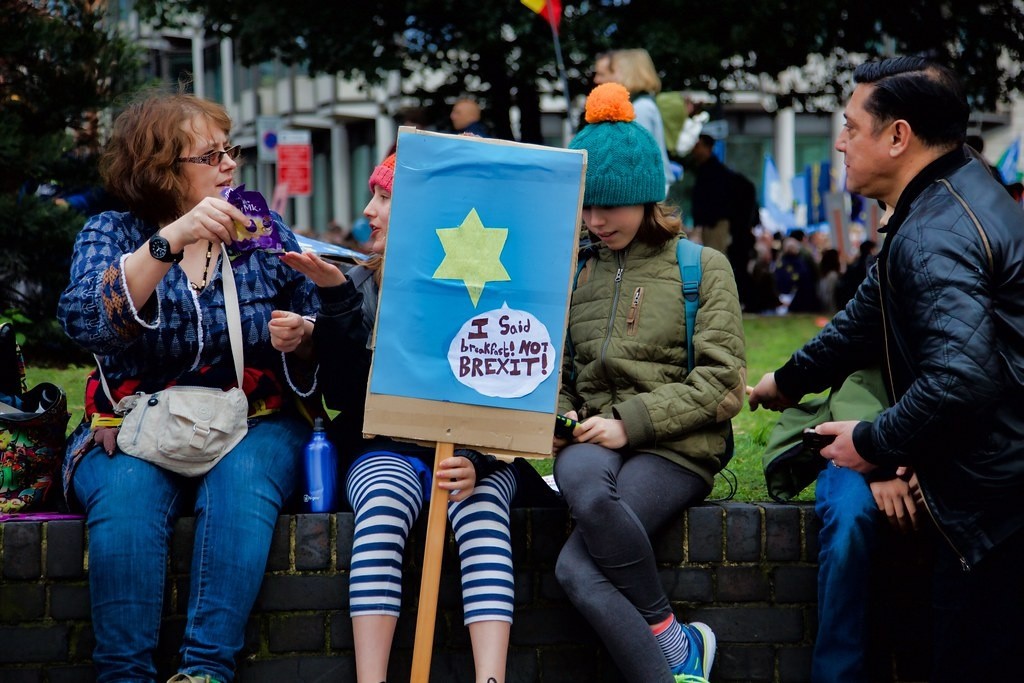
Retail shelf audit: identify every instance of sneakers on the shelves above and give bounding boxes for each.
[674,621,717,679]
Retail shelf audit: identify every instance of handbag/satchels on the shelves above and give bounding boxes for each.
[0,381,69,514]
[110,383,250,478]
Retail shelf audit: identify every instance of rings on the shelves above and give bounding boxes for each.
[832,459,842,469]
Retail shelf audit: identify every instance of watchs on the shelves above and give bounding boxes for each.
[148,227,185,265]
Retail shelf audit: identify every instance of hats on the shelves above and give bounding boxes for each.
[369,154,395,195]
[565,80,665,207]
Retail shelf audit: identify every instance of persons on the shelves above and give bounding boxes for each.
[552,83,744,683]
[327,98,485,253]
[748,57,1024,683]
[592,46,878,318]
[277,154,524,683]
[58,95,318,683]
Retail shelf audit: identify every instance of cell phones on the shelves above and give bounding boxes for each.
[801,428,837,455]
[555,414,583,440]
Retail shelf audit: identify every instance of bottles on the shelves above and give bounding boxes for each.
[302,418,339,513]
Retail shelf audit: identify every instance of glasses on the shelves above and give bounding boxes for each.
[178,144,243,166]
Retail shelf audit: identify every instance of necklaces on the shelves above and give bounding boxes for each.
[189,241,213,295]
